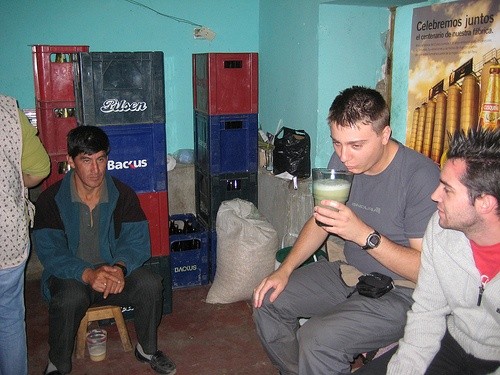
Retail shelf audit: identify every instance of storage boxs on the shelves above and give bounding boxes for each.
[31,44,259,328]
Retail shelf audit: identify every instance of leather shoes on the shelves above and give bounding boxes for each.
[134,344,177,375]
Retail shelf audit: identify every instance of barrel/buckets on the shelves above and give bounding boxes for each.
[274,245,329,326]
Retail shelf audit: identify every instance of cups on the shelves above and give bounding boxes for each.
[312,168,353,226]
[87,329,107,361]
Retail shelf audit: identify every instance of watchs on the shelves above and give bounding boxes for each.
[355,229,384,249]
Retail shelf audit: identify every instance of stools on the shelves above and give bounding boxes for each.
[75,305,134,359]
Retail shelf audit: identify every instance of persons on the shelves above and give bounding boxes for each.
[249,86,443,375]
[386,127,499,375]
[25,125,176,375]
[1,91,53,375]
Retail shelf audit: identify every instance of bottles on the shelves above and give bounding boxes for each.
[56,53,72,62]
[56,109,76,118]
[227,180,240,190]
[59,162,71,174]
[477,66,500,131]
[169,218,200,252]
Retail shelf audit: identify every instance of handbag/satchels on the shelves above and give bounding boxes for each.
[272,127,312,178]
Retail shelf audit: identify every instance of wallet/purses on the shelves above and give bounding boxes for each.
[357,271,395,299]
